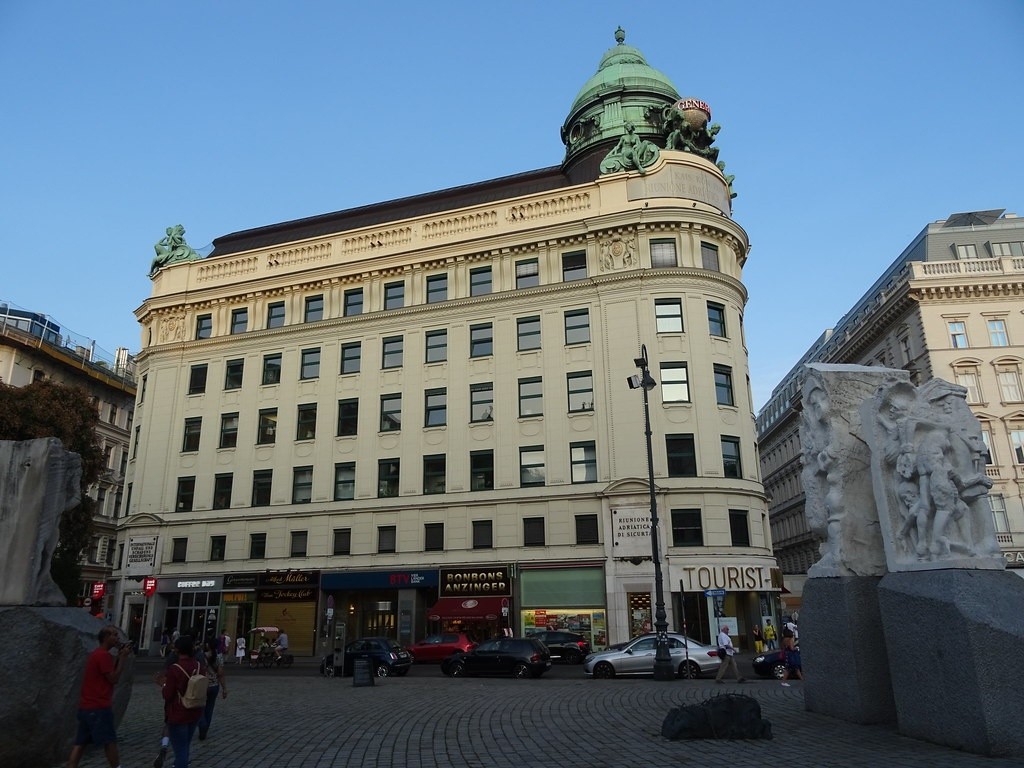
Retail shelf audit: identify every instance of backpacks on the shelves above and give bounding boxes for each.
[172,662,208,709]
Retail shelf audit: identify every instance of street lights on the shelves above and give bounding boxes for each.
[625,342,676,682]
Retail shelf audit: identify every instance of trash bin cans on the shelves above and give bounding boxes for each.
[353,656,374,687]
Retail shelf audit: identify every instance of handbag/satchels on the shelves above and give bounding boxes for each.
[717,648,726,660]
[779,651,785,659]
[772,625,777,641]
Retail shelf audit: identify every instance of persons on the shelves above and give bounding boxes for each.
[147,614,233,768]
[546,622,559,632]
[596,629,606,646]
[145,224,174,277]
[631,620,651,639]
[748,617,805,684]
[65,625,135,767]
[236,633,246,665]
[607,123,659,174]
[274,627,288,661]
[577,642,590,654]
[714,624,748,684]
[665,120,738,200]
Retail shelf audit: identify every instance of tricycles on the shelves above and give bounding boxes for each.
[245,626,295,670]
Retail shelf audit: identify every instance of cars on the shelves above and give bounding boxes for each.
[525,630,591,665]
[440,636,552,680]
[753,637,802,681]
[583,631,722,680]
[318,637,414,677]
[407,632,479,664]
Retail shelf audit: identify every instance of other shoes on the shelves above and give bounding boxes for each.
[737,678,747,683]
[781,682,791,687]
[716,680,725,684]
[154,745,169,768]
[276,656,281,661]
